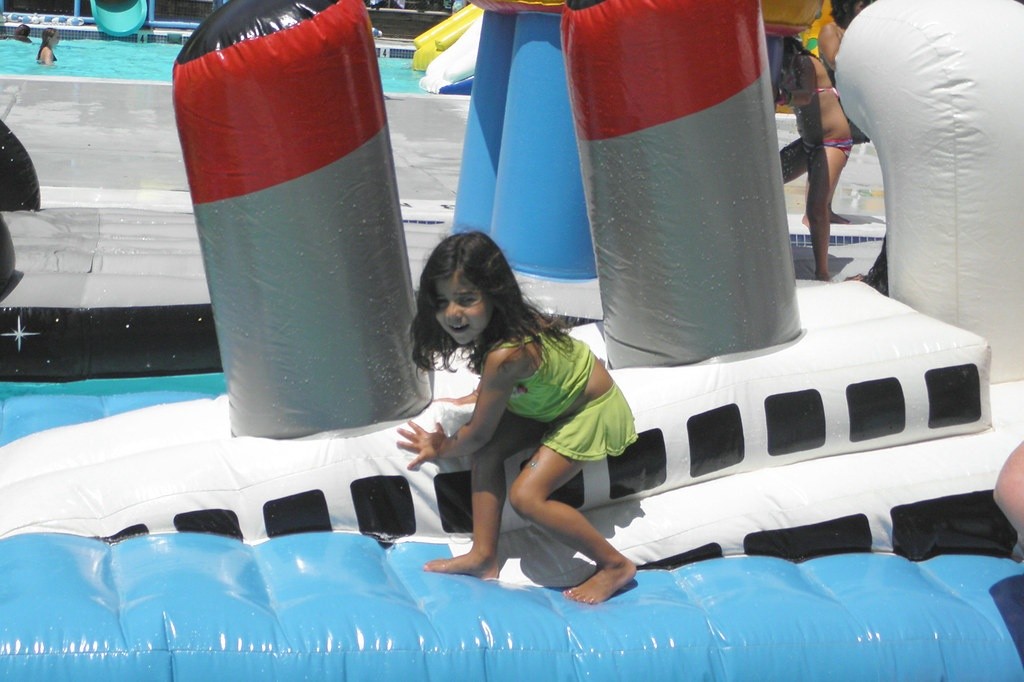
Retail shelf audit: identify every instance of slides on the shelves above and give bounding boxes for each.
[89,1,149,38]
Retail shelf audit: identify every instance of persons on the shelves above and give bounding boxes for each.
[993,440,1024,537]
[396,232,639,604]
[14,24,32,43]
[36,28,59,65]
[766,0,895,281]
